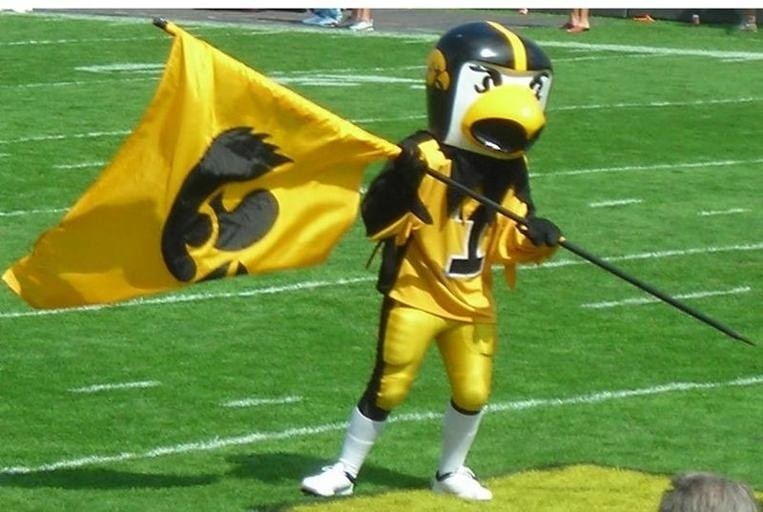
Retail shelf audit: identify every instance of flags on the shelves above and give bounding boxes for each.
[0,20,403,312]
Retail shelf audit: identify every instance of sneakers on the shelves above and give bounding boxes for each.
[430,460,493,502]
[300,461,358,499]
[298,14,374,34]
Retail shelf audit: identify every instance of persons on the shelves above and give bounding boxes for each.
[302,8,343,28]
[298,20,569,508]
[338,8,374,32]
[560,8,590,33]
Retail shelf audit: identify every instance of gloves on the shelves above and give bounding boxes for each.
[522,215,562,247]
[392,140,430,191]
